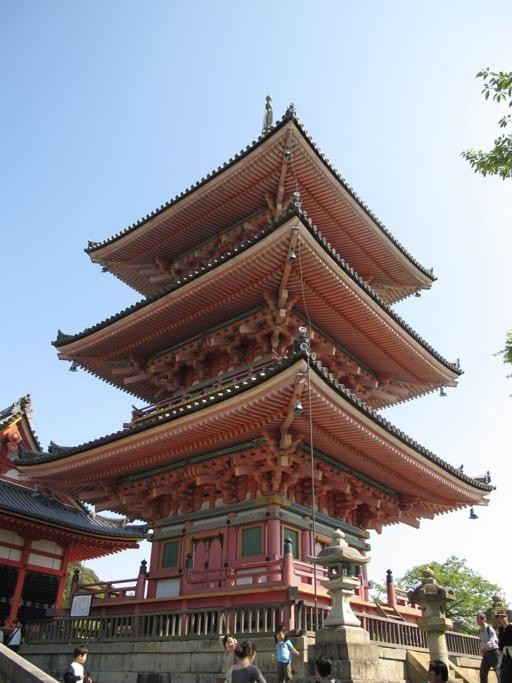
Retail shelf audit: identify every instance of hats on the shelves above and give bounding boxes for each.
[494,611,507,617]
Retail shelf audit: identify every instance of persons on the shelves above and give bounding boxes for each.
[427,660,448,683]
[475,612,500,683]
[273,624,299,682]
[315,654,337,682]
[224,639,267,682]
[494,609,512,683]
[219,634,256,683]
[6,618,27,652]
[64,645,93,683]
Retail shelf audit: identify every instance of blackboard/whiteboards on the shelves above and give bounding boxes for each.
[69,594,93,617]
